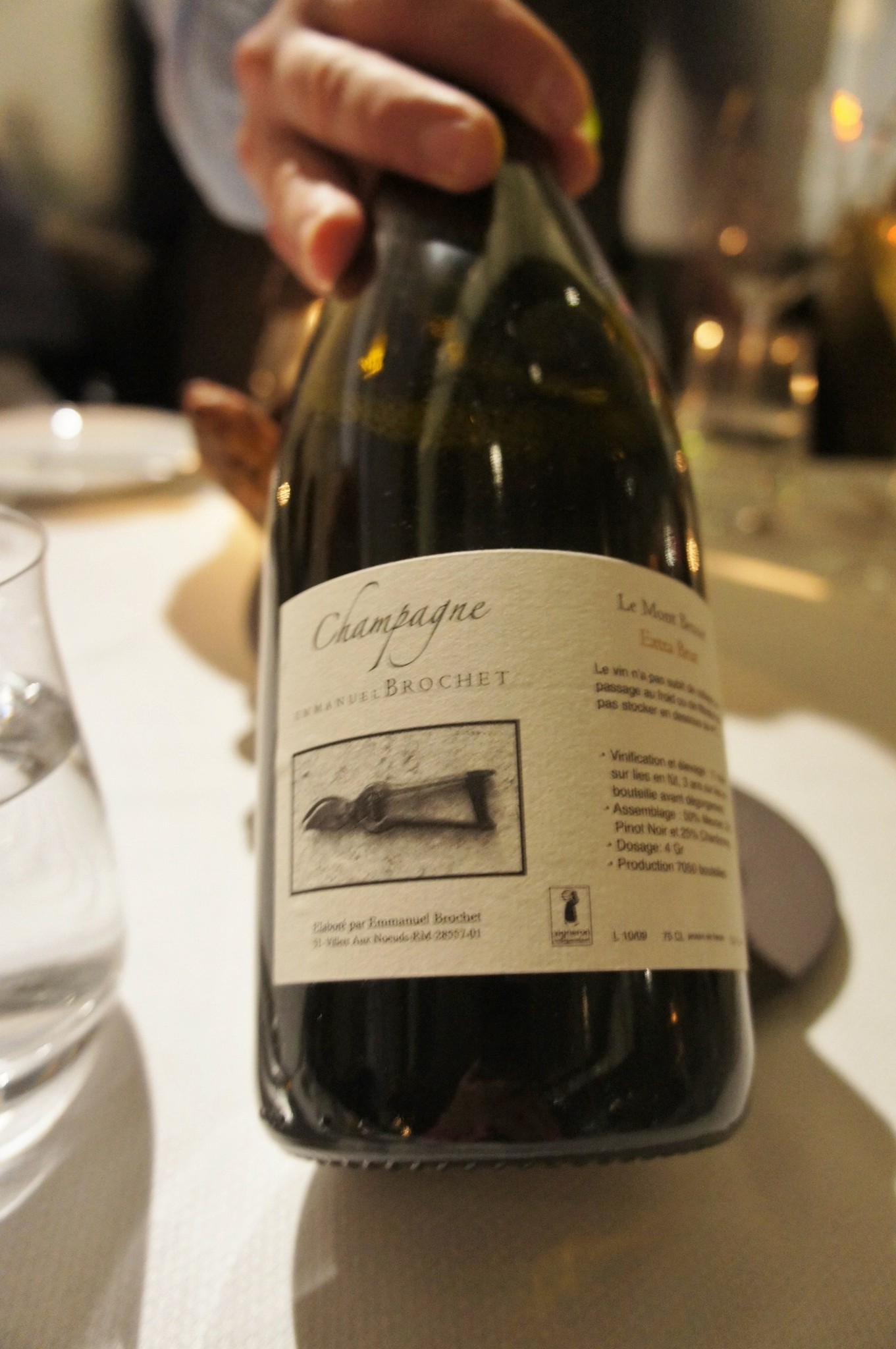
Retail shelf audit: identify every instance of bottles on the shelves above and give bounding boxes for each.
[254,79,760,1175]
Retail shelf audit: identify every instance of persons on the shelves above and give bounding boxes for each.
[140,0,597,295]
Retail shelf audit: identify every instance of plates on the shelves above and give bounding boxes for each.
[0,401,205,497]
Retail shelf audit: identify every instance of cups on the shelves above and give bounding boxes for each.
[1,501,136,1222]
